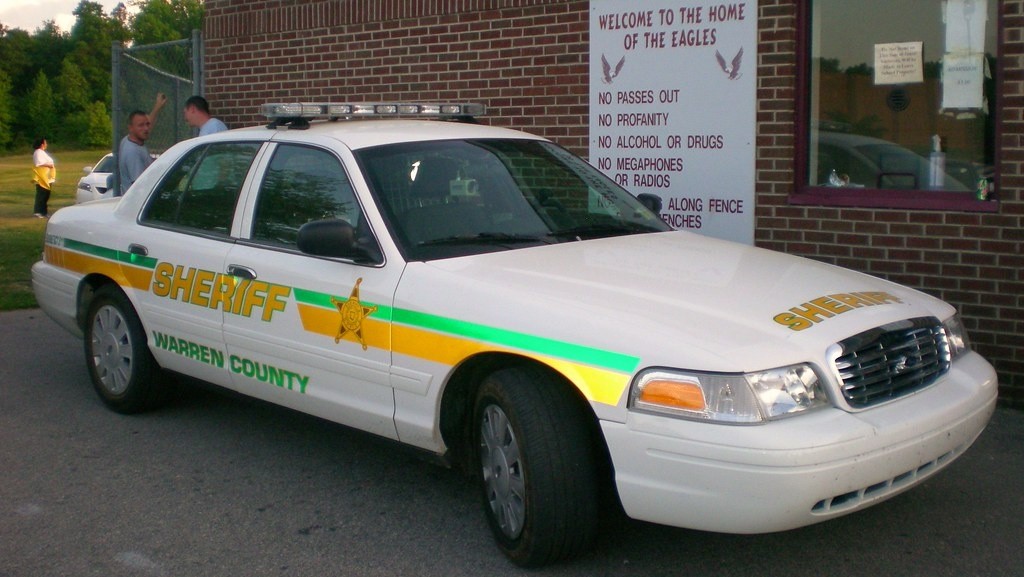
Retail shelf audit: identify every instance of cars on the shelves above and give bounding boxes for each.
[816,119,970,192]
[76,153,160,203]
[32,103,999,569]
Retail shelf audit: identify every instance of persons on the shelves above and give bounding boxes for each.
[118,92,168,196]
[31,137,56,219]
[186,96,234,190]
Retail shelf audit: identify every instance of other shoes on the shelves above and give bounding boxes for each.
[34,211,52,218]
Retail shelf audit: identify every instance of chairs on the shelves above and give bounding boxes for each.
[399,159,466,241]
